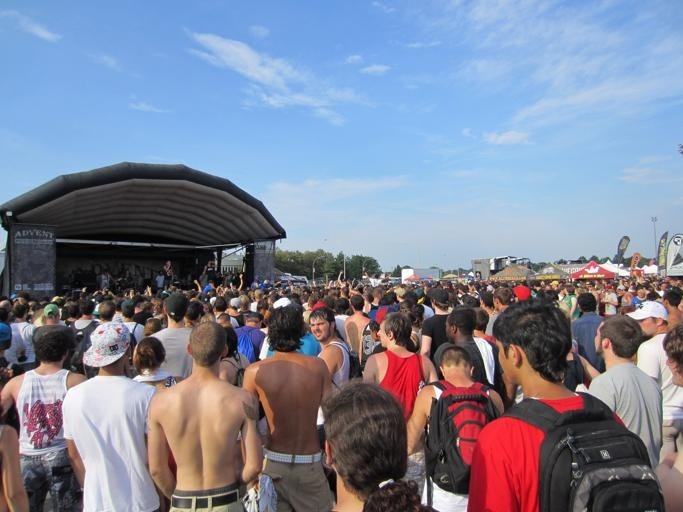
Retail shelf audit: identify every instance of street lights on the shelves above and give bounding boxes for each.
[650,216,658,256]
[311,256,327,281]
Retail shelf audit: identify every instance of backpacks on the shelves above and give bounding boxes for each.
[329,342,361,391]
[419,381,500,507]
[500,391,665,512]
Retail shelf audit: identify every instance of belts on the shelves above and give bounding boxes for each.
[171,492,236,509]
[262,446,322,464]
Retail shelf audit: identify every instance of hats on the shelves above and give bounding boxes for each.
[512,286,530,302]
[166,294,187,315]
[624,301,668,326]
[43,303,59,317]
[81,322,131,368]
[0,321,11,343]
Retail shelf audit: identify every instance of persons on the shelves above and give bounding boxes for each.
[0,259,683,512]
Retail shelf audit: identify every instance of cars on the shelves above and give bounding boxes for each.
[274,267,308,287]
